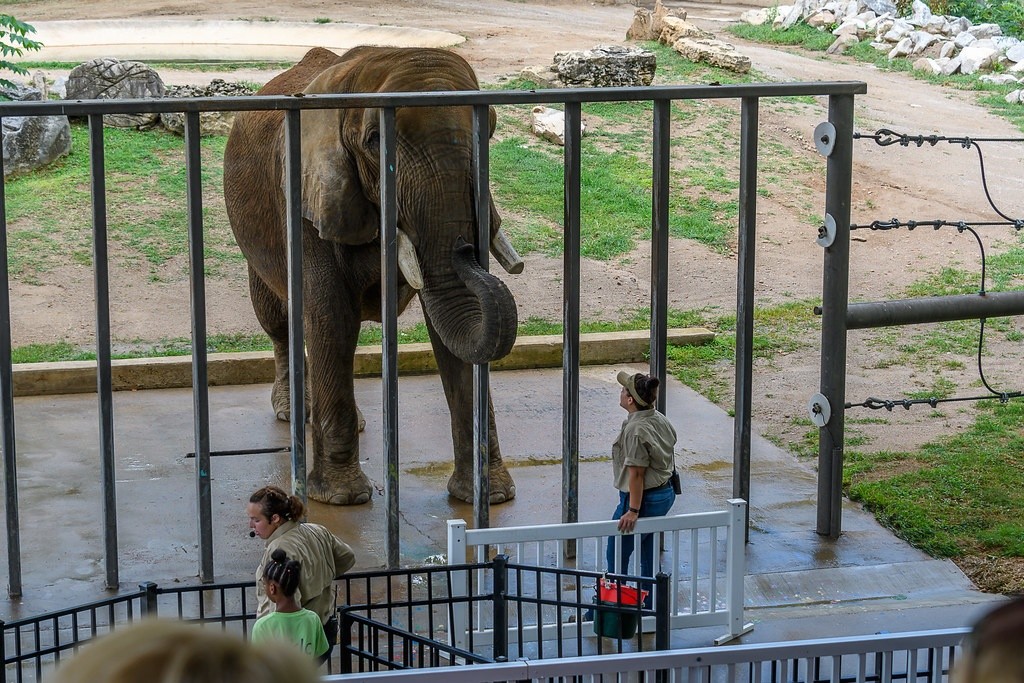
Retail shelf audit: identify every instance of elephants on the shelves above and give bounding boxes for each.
[222,47,519,506]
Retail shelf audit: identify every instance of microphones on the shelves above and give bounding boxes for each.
[249,530,255,537]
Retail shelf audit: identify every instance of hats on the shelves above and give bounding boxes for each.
[617,371,656,407]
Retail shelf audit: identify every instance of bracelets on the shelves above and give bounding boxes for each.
[629,507,639,513]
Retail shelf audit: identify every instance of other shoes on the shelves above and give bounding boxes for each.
[568,616,586,623]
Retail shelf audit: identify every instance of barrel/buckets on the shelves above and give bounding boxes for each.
[592,595,640,639]
[592,577,649,605]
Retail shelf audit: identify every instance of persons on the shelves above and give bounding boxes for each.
[568,372,677,623]
[43,486,355,683]
[949,598,1024,683]
